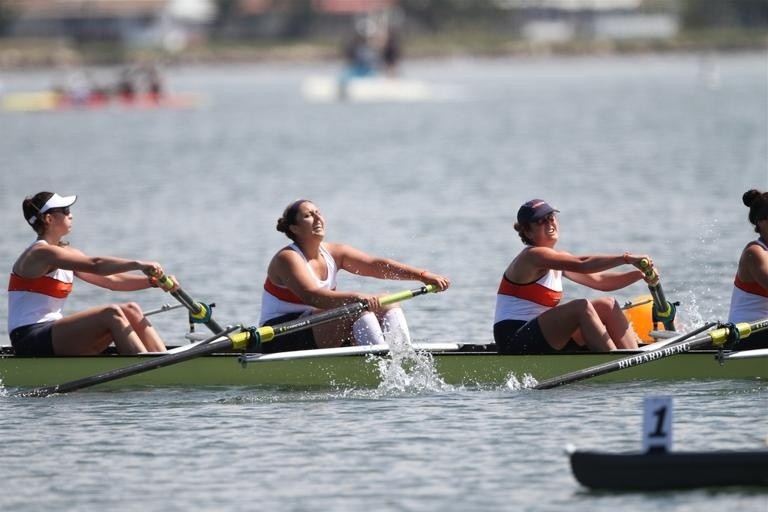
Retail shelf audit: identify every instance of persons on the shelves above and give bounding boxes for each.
[338,9,407,101]
[47,54,168,109]
[492,198,661,352]
[8,190,183,357]
[257,199,452,351]
[724,188,767,351]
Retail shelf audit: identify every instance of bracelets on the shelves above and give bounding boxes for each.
[417,270,429,282]
[623,251,631,264]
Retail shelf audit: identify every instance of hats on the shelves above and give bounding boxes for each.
[518,200,557,219]
[28,193,78,226]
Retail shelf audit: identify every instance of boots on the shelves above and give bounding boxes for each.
[352,312,384,345]
[384,307,410,346]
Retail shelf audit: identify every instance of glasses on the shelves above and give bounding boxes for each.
[51,208,70,216]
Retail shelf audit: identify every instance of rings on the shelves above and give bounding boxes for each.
[444,279,449,284]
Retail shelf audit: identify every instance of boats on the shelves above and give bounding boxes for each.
[300,74,429,101]
[2,89,205,116]
[1,329,768,399]
[561,444,767,497]
